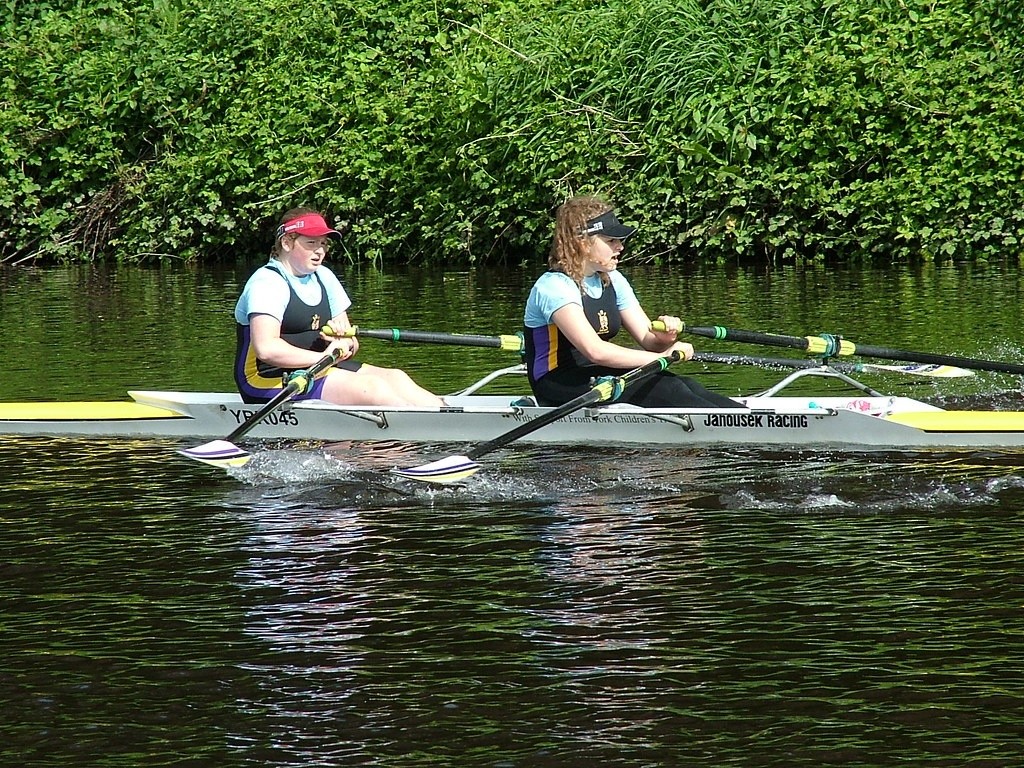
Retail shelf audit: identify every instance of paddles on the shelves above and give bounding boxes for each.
[389,351,685,484]
[649,319,1024,378]
[322,324,976,380]
[175,346,345,471]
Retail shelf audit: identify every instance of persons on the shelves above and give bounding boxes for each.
[234,207,450,407]
[523,196,749,408]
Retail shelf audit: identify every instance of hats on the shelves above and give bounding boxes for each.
[274,213,343,240]
[577,210,637,240]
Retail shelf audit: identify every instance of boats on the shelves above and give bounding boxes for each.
[0,387,1024,451]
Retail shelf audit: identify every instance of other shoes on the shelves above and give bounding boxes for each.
[511,395,538,407]
[846,400,871,411]
